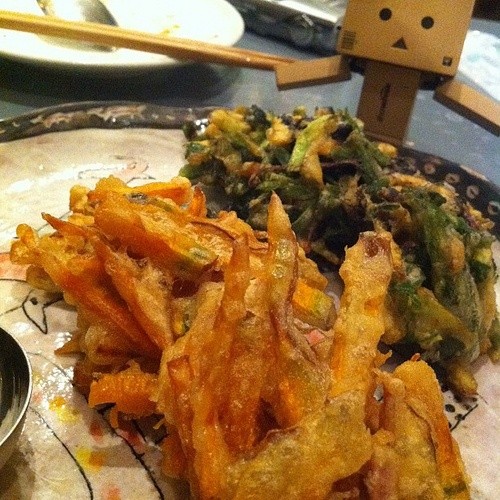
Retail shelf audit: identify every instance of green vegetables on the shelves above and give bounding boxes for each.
[172,99,500,401]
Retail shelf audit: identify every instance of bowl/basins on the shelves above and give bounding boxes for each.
[0,327,33,469]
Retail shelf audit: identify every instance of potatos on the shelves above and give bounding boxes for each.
[9,174,472,500]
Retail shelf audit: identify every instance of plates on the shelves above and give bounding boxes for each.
[0,99,500,500]
[0,0,244,80]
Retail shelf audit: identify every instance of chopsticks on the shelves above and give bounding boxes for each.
[0,9,298,71]
